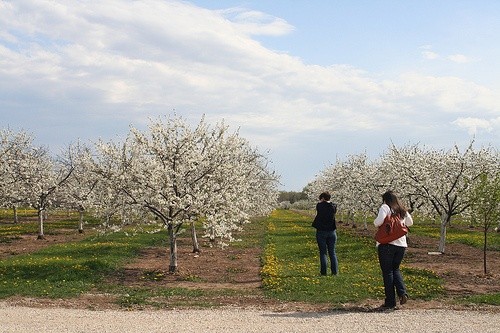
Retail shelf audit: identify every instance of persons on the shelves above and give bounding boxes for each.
[373,190,414,310]
[311,190,338,275]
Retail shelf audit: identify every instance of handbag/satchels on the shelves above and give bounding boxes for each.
[375,202,411,244]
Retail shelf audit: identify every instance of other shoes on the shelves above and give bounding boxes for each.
[399,293,408,305]
